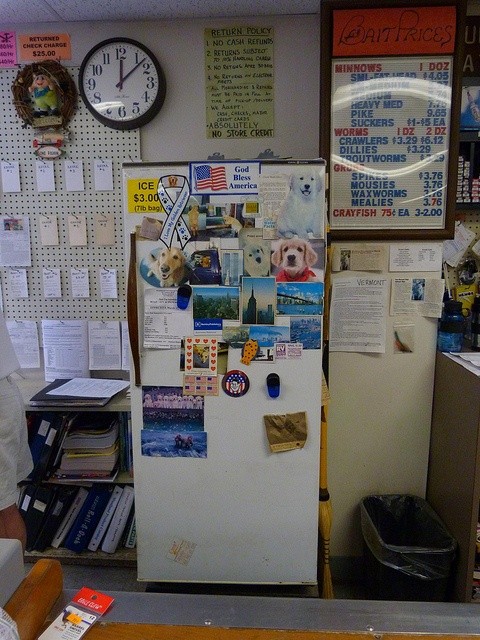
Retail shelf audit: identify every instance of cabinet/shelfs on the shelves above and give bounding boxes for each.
[15,398,136,562]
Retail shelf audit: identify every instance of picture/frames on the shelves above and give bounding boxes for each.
[319,4,465,242]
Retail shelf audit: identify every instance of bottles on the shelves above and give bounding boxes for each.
[437,300,464,353]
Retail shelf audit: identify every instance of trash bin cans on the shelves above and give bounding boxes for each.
[360,493,459,602]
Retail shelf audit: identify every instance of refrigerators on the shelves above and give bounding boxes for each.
[121,159,323,582]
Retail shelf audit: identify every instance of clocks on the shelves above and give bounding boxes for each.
[79,37,166,130]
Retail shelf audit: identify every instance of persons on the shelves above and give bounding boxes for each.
[0,312,35,558]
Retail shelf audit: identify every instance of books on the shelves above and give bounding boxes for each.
[16,412,137,482]
[29,378,124,408]
[17,482,136,554]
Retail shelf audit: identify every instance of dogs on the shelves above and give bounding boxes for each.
[274,166,323,240]
[270,237,320,282]
[242,240,271,278]
[193,345,209,363]
[144,248,187,288]
[142,393,202,409]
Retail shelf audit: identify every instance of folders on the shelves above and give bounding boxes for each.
[19,486,35,521]
[26,415,55,479]
[34,425,58,480]
[35,487,78,553]
[25,485,51,551]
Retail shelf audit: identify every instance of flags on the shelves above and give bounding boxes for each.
[211,167,227,190]
[195,165,213,189]
